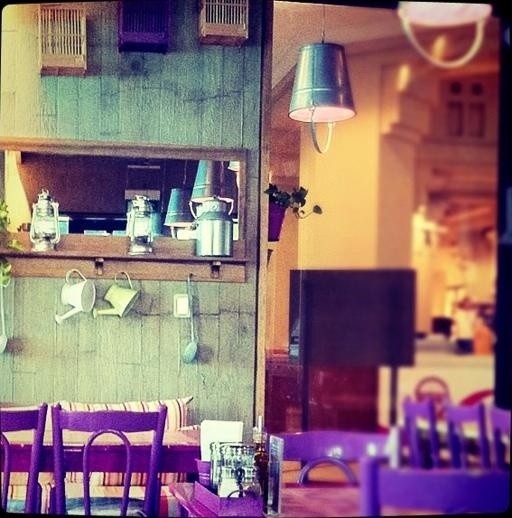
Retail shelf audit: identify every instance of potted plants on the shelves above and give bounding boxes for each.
[264,184,322,241]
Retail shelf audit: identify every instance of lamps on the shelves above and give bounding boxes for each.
[29,189,61,253]
[164,159,199,239]
[289,6,356,156]
[126,195,154,255]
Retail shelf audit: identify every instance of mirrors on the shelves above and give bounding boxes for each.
[0,137,250,283]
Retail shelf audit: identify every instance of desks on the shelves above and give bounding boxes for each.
[0,430,200,473]
[398,419,512,461]
[169,483,443,518]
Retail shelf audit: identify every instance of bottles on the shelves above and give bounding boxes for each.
[216,466,262,499]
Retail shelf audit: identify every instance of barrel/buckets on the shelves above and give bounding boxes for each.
[163,186,195,240]
[290,41,357,155]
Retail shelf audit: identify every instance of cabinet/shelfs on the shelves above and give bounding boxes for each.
[265,349,379,434]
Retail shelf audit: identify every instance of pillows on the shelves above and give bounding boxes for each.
[0,395,200,486]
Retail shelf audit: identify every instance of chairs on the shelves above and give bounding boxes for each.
[357,456,511,517]
[0,402,47,518]
[283,431,390,487]
[402,396,512,469]
[51,402,167,518]
